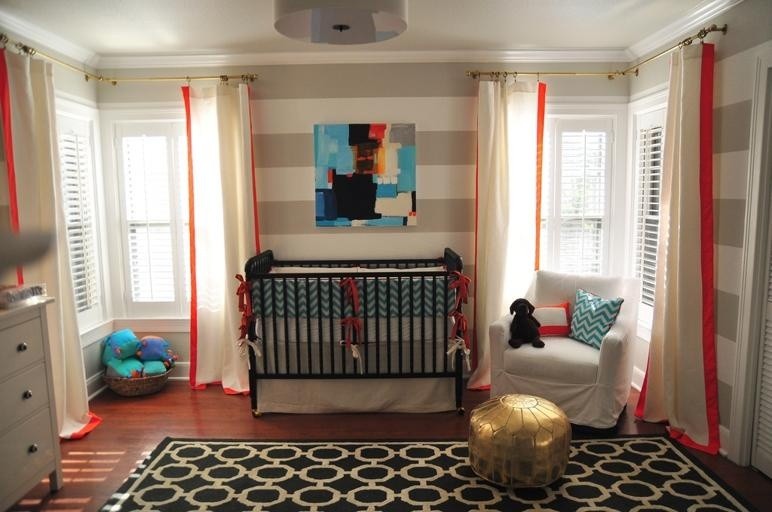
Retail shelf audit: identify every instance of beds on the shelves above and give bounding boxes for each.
[243,247,467,417]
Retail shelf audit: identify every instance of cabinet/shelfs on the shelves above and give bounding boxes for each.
[0,296,63,512]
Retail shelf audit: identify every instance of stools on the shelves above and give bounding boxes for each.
[468,392,572,489]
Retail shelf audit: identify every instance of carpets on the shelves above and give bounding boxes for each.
[101,431,754,512]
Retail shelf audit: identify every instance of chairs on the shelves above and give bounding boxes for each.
[488,268,643,429]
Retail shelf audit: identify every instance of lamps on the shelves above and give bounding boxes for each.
[272,0,410,45]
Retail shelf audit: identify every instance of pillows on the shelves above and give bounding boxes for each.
[533,300,571,337]
[570,286,623,349]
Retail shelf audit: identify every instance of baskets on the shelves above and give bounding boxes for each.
[102,367,173,396]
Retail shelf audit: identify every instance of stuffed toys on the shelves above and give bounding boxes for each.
[508,298,545,349]
